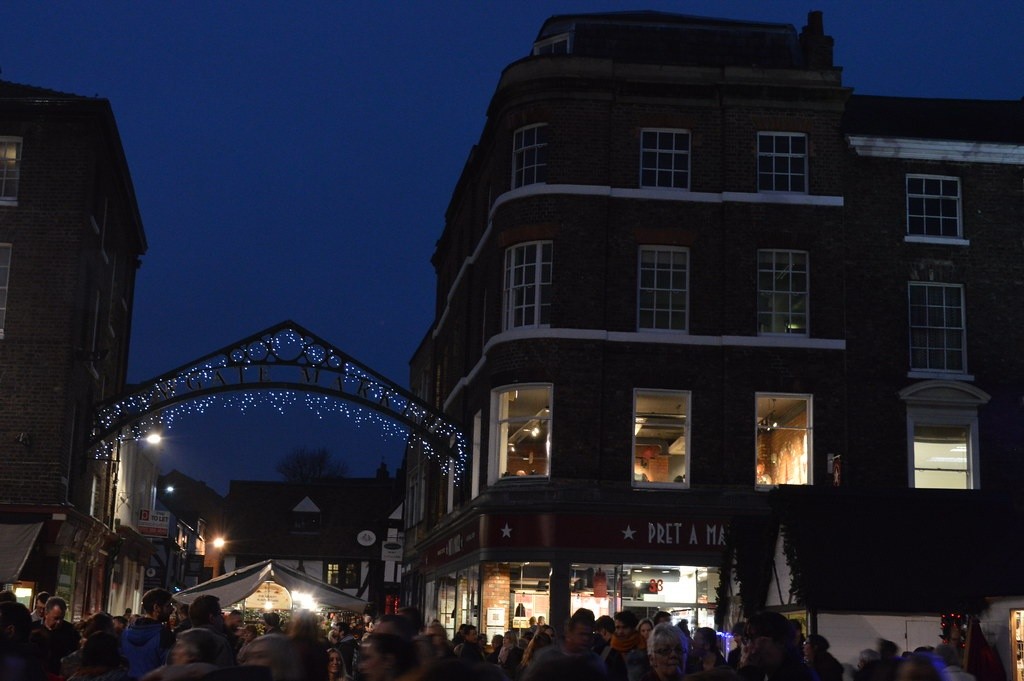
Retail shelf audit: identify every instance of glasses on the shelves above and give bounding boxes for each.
[803,641,811,646]
[653,647,684,656]
[741,633,759,645]
[220,612,224,616]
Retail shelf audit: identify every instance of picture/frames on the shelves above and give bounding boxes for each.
[485,627,504,646]
[487,608,504,626]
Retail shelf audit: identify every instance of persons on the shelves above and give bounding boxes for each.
[0,588,977,681]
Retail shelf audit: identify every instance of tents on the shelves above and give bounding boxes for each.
[172,560,366,615]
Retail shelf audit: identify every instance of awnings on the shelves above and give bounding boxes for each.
[0,521,44,583]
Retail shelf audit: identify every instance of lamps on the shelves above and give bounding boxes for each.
[761,422,770,431]
[769,417,778,427]
[534,422,541,433]
